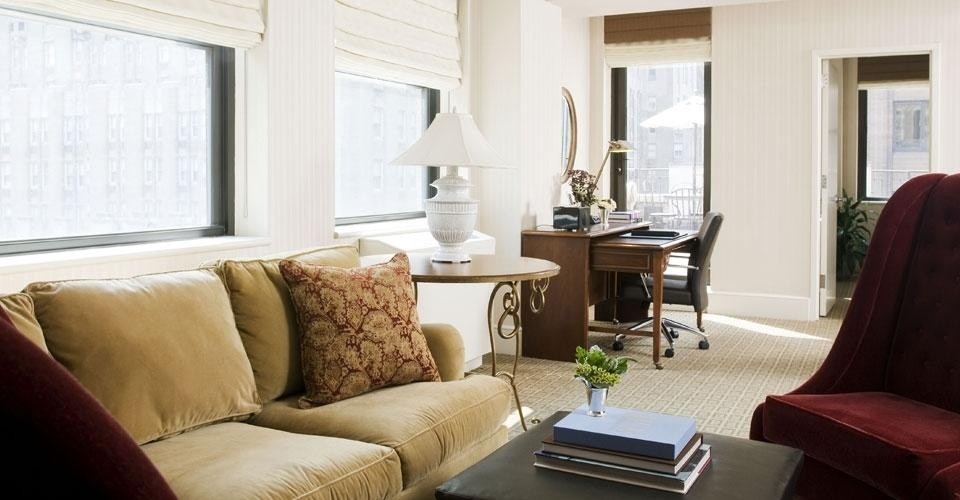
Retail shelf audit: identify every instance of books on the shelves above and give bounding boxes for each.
[533,403,713,496]
[607,211,641,221]
[608,218,643,224]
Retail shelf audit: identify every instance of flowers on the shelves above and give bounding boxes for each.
[597,199,612,208]
[573,344,640,389]
[566,168,598,205]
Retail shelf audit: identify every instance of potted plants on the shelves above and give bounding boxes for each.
[838,188,871,282]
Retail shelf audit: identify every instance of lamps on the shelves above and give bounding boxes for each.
[594,140,636,189]
[388,113,518,262]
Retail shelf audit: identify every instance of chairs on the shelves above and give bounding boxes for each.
[612,211,724,357]
[748,174,960,500]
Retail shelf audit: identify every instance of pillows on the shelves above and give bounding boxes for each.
[1,294,49,356]
[22,266,263,442]
[201,244,361,403]
[279,249,442,408]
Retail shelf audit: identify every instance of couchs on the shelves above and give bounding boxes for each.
[0,236,507,497]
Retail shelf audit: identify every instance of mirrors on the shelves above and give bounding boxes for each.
[560,87,578,185]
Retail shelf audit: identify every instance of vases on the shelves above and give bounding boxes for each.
[586,387,609,416]
[600,208,608,225]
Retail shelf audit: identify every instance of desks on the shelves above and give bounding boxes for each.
[362,255,562,431]
[521,222,698,369]
